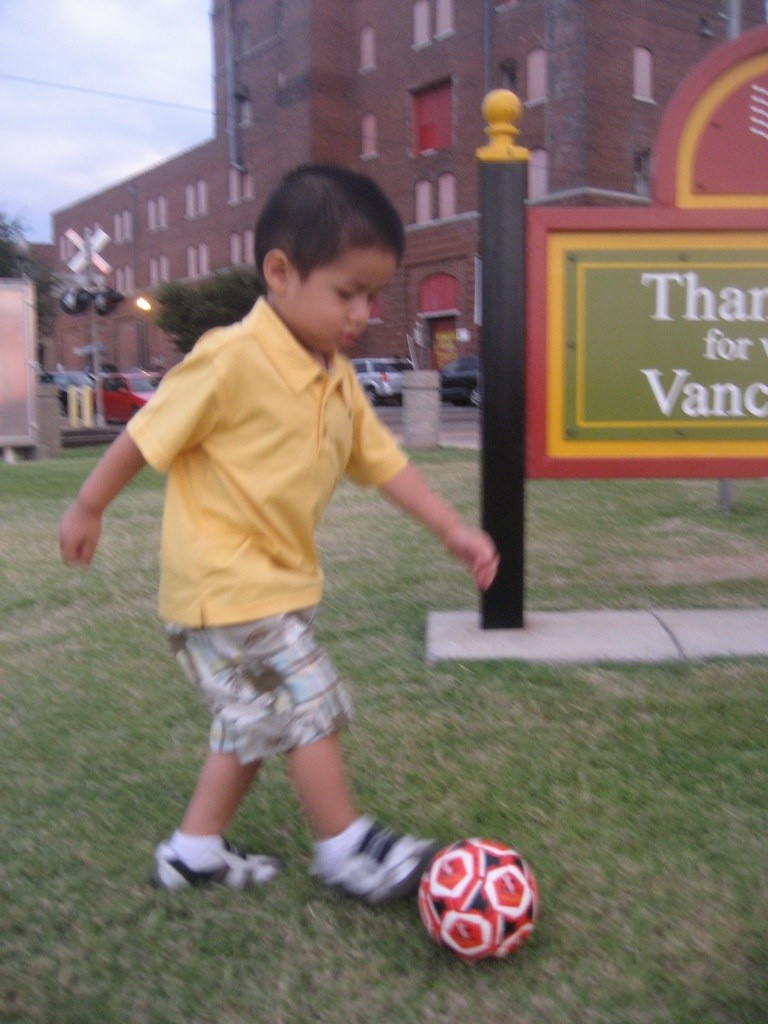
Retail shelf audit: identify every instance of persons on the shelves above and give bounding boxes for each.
[59,162,501,909]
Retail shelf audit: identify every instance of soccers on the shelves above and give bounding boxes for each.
[419,836,540,963]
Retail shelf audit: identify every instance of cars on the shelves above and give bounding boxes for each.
[439,355,480,407]
[42,368,98,419]
[349,357,417,406]
[100,371,158,426]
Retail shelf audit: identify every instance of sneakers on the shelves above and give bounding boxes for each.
[154,830,284,895]
[307,821,434,906]
